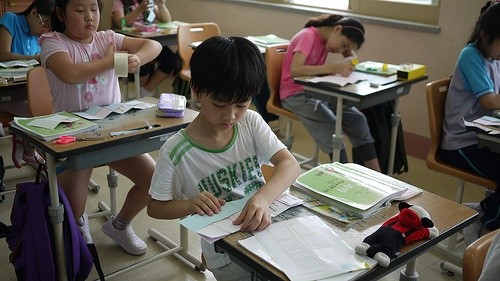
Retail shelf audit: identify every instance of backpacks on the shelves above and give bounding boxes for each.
[0,161,105,281]
[363,98,409,174]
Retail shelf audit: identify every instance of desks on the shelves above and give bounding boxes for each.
[0,22,500,281]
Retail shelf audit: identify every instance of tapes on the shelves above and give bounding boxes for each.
[113,52,128,78]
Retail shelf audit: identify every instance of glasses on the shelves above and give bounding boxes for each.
[39,14,48,33]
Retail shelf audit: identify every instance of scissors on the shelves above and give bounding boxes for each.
[54,135,105,144]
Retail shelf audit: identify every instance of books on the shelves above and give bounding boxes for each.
[0,60,41,81]
[12,111,96,141]
[354,60,399,77]
[239,215,377,281]
[244,34,288,49]
[291,159,424,224]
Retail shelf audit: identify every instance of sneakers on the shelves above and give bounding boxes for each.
[76,210,94,245]
[22,151,47,171]
[12,134,26,168]
[102,214,147,256]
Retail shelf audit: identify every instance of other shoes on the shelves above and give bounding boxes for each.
[460,201,485,247]
[140,86,157,99]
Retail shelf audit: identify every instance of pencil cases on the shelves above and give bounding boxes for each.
[156,93,186,118]
[133,20,157,32]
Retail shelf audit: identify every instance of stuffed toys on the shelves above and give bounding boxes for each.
[352,202,442,267]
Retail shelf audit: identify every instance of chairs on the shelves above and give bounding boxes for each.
[265,45,299,149]
[27,67,120,221]
[177,23,219,82]
[424,79,497,261]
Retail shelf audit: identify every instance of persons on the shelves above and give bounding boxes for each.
[440,0,500,246]
[110,0,178,97]
[0,0,57,169]
[279,14,381,173]
[37,0,164,258]
[146,35,302,281]
